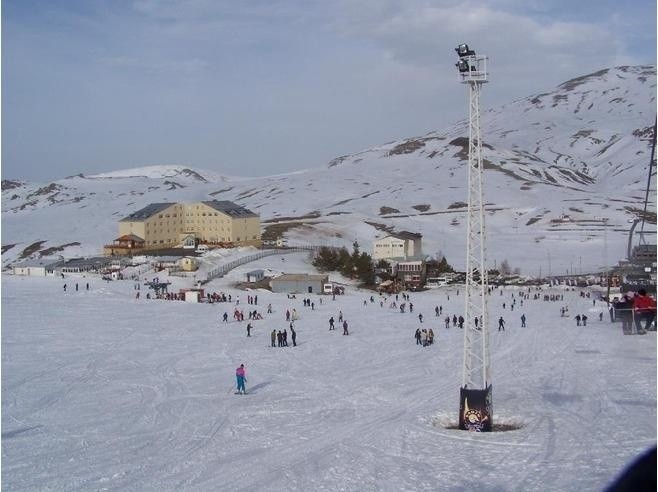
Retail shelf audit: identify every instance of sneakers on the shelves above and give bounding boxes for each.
[624,329,648,335]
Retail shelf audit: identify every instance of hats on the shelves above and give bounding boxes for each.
[638,289,646,296]
[627,291,634,299]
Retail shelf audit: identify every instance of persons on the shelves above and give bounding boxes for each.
[207,292,232,304]
[320,298,322,304]
[339,311,343,322]
[223,312,228,321]
[303,298,314,310]
[233,308,243,320]
[236,296,240,304]
[271,309,296,347]
[62,273,64,278]
[133,282,184,300]
[267,304,273,313]
[87,282,89,289]
[249,310,262,320]
[287,293,295,299]
[364,285,603,347]
[333,292,335,300]
[329,317,335,330]
[64,284,66,291]
[76,283,78,290]
[343,320,349,335]
[236,363,247,395]
[248,295,257,305]
[247,324,253,336]
